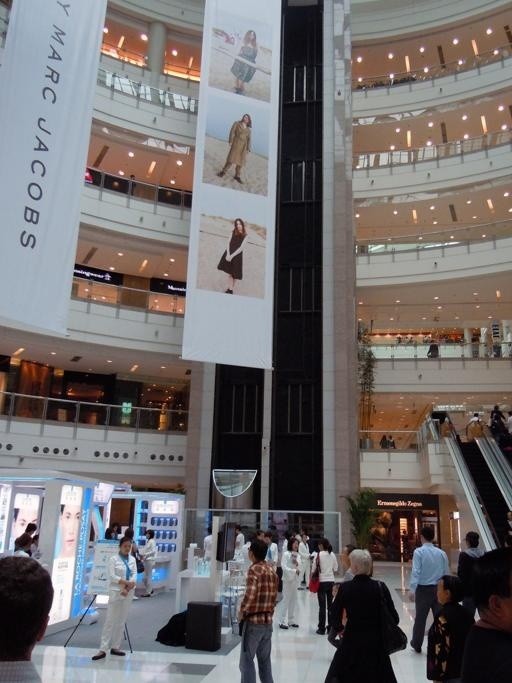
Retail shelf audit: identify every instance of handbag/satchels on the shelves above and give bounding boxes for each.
[279,624,288,629]
[387,625,407,655]
[316,629,325,635]
[136,558,144,573]
[289,624,299,627]
[309,567,320,593]
[327,629,331,633]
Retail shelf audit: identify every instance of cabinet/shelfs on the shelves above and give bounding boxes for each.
[177,569,210,613]
[146,498,183,590]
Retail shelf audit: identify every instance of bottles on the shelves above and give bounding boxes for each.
[193,555,209,577]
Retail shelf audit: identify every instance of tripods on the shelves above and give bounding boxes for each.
[63,594,133,653]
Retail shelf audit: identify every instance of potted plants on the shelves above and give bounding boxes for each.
[358,349,376,450]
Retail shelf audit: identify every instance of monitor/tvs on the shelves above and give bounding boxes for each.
[216,522,236,562]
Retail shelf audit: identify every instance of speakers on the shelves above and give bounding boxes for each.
[186,601,222,651]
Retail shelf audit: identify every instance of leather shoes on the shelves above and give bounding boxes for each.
[410,640,421,653]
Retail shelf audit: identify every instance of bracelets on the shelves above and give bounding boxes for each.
[126,587,130,592]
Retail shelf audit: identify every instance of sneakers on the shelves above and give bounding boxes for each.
[141,594,150,597]
[151,590,154,593]
[92,652,106,660]
[110,648,125,656]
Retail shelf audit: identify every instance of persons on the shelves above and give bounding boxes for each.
[90,536,138,661]
[322,545,399,683]
[410,527,512,682]
[14,522,39,556]
[395,334,432,344]
[104,522,157,600]
[468,404,512,443]
[235,523,339,635]
[379,435,397,450]
[229,29,258,94]
[0,556,54,683]
[55,503,81,560]
[402,530,410,562]
[128,173,138,196]
[12,497,39,539]
[217,113,253,185]
[426,339,439,358]
[239,539,281,683]
[216,218,248,294]
